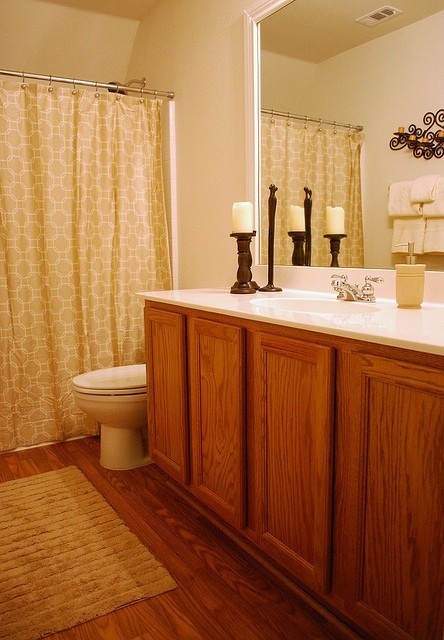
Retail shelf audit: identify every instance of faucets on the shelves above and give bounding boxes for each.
[332,275,382,302]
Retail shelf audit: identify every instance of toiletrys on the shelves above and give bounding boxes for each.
[395,240,426,310]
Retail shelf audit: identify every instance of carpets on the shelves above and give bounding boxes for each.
[0,465,177,639]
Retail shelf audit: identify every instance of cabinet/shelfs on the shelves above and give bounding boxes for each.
[143,294,442,640]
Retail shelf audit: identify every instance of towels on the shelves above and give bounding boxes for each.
[387,175,443,255]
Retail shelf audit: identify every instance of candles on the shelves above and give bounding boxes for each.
[438,130,444,137]
[288,205,306,233]
[323,204,345,234]
[409,135,415,140]
[230,200,254,235]
[397,126,405,133]
[421,137,428,141]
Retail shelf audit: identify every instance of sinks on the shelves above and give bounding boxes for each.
[248,294,382,315]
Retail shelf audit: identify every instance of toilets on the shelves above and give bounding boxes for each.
[73,363,151,469]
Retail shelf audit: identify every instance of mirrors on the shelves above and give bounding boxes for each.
[241,2,444,305]
